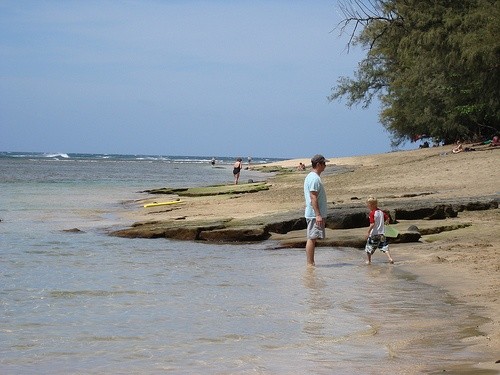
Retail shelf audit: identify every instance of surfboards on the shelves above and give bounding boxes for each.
[144,200,182,208]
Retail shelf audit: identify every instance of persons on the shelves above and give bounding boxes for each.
[419,135,500,154]
[305,154,329,269]
[233,158,243,184]
[364,197,395,264]
[297,162,305,171]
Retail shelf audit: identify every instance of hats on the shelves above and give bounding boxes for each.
[311,154,330,162]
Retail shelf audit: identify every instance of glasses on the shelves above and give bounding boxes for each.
[319,162,325,165]
